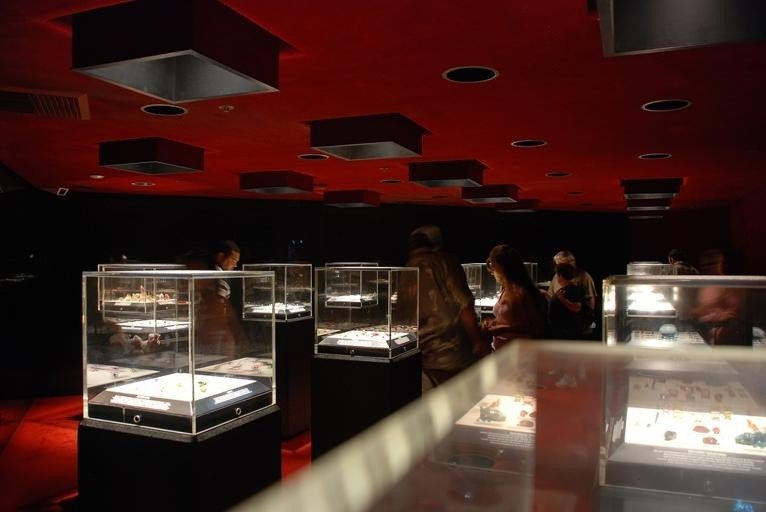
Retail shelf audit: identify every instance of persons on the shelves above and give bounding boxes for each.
[548,261,587,336]
[687,254,766,351]
[395,225,491,388]
[550,248,598,311]
[668,247,702,307]
[484,242,549,351]
[186,239,253,362]
